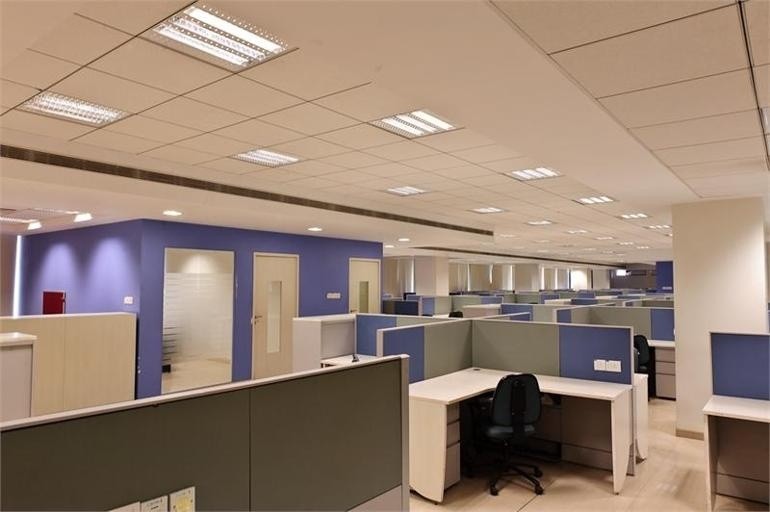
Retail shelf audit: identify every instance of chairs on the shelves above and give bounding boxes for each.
[484,373,547,498]
[632,333,654,402]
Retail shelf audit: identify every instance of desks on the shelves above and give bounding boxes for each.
[633,373,649,460]
[409,368,634,504]
[648,339,676,401]
[321,354,377,366]
[699,329,770,510]
